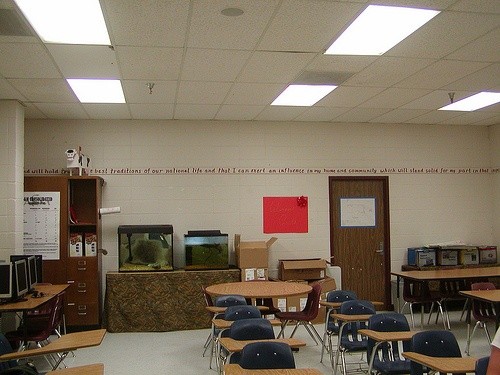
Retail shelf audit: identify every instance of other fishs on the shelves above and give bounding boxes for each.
[147,262,162,270]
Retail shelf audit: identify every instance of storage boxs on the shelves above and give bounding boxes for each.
[235,235,278,281]
[408,245,497,267]
[272,293,308,312]
[309,276,336,295]
[280,258,331,280]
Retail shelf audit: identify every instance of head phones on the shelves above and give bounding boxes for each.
[31,291,44,298]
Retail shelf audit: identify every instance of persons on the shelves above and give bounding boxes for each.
[487,326,500,375]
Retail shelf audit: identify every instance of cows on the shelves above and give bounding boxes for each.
[64,148,92,177]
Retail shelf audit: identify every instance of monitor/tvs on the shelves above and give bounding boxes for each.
[0,262,12,304]
[10,255,43,283]
[27,256,37,293]
[15,259,28,301]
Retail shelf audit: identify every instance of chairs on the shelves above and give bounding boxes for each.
[200,266,497,375]
[6,292,76,370]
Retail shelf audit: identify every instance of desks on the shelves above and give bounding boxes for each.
[0,284,70,311]
[205,282,312,306]
[106,271,240,333]
[390,266,500,313]
[459,289,500,355]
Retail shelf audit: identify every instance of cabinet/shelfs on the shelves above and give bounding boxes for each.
[23,175,103,326]
